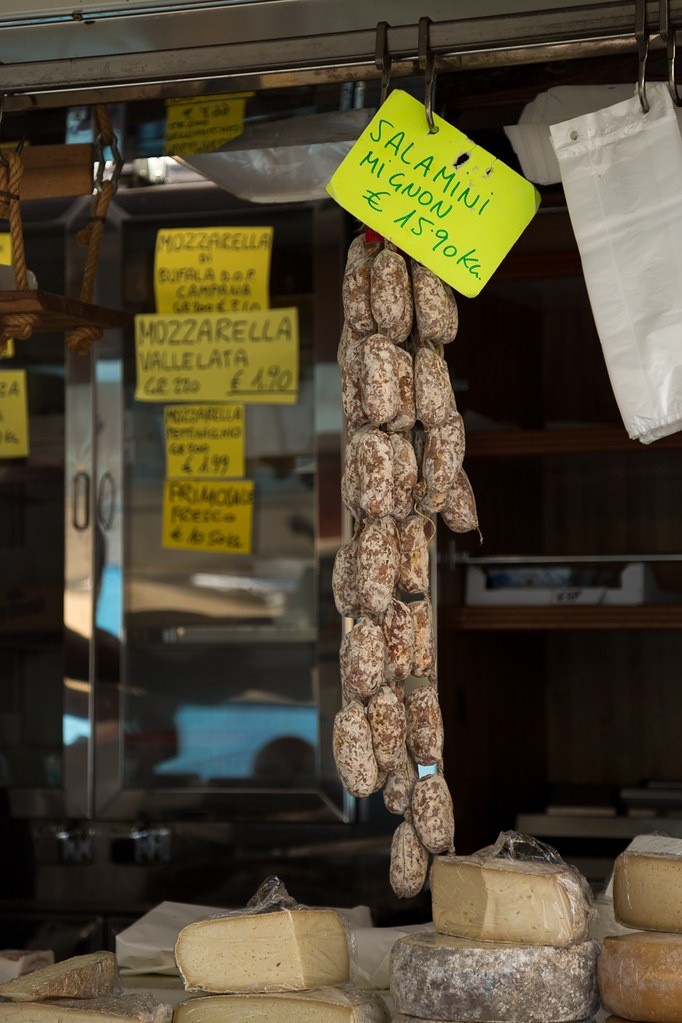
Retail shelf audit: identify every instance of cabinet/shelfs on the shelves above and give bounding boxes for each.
[448,253,682,630]
[0,182,381,831]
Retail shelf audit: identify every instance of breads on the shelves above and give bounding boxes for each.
[597,834,681,1023]
[391,855,601,1021]
[175,908,391,1023]
[1,949,173,1023]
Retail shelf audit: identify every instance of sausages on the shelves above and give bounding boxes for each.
[331,234,480,895]
